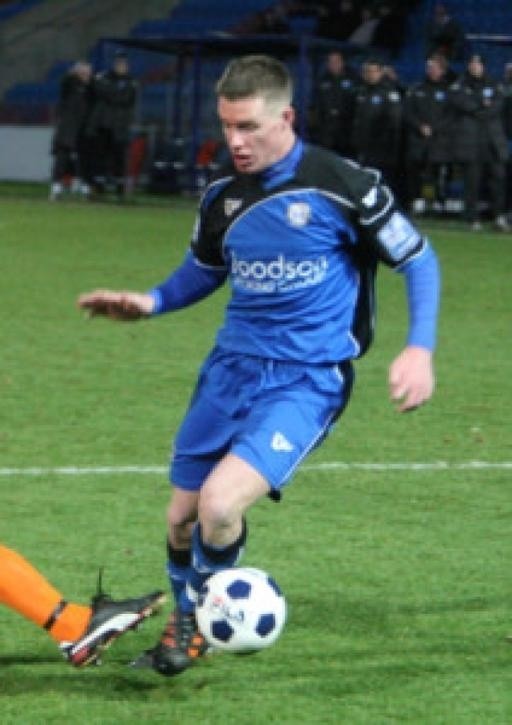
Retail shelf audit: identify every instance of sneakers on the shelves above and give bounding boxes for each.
[57,590,168,669]
[148,610,211,677]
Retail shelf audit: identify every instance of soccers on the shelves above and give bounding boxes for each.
[193,564,287,655]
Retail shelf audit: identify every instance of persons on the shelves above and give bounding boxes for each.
[76,55,440,677]
[0,545,167,670]
[50,58,137,193]
[313,53,511,228]
[255,0,466,62]
